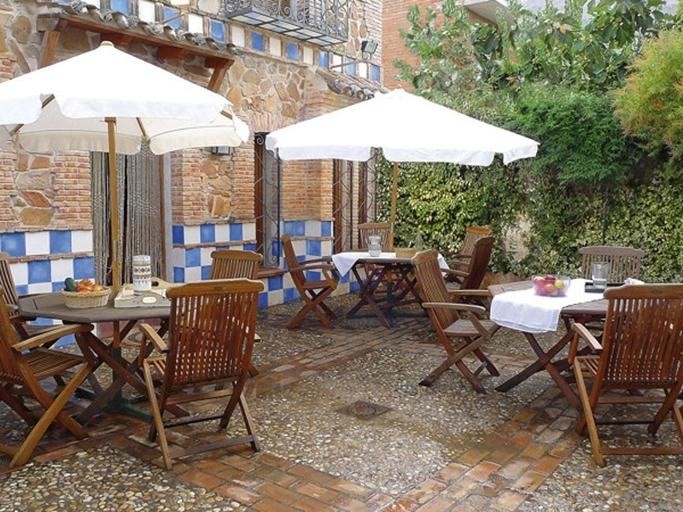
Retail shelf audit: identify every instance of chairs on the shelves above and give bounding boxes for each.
[128,249,265,469]
[0,250,93,467]
[356,221,400,292]
[280,234,339,329]
[409,227,503,393]
[560,244,682,466]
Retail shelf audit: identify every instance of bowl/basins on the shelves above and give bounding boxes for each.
[532,274,571,296]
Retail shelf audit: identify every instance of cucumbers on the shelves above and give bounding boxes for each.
[65,277,77,291]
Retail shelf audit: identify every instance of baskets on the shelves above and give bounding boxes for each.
[62,288,111,308]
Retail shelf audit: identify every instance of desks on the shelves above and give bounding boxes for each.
[331,249,442,328]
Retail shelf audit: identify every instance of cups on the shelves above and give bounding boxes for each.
[132,255,151,291]
[591,262,609,290]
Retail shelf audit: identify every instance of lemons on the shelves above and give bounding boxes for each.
[555,279,564,288]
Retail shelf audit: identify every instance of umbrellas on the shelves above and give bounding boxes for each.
[0,40,252,402]
[261,80,542,302]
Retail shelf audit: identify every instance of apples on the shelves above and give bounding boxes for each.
[532,274,558,296]
[92,284,103,291]
